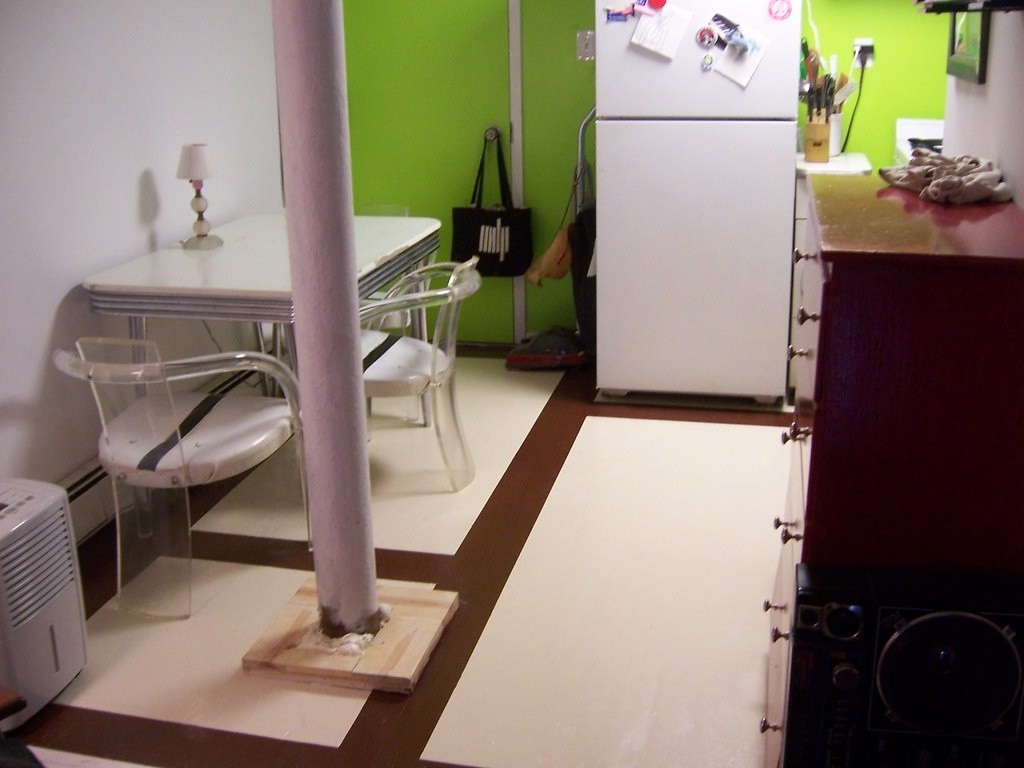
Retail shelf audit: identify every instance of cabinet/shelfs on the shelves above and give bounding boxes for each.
[762,174,1024,768]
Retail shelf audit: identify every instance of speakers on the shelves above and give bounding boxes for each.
[783,563,1024,768]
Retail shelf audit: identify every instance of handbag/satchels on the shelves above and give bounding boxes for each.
[451,128,533,278]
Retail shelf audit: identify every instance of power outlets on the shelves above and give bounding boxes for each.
[854,38,874,67]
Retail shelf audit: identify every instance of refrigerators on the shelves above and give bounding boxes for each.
[594,0,803,412]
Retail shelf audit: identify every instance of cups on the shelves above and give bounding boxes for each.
[829,113,843,156]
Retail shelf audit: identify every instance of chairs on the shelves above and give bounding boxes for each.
[54,338,314,621]
[359,256,483,492]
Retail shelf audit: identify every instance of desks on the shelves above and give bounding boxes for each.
[84,212,442,551]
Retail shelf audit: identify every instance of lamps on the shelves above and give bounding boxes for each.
[177,143,224,250]
